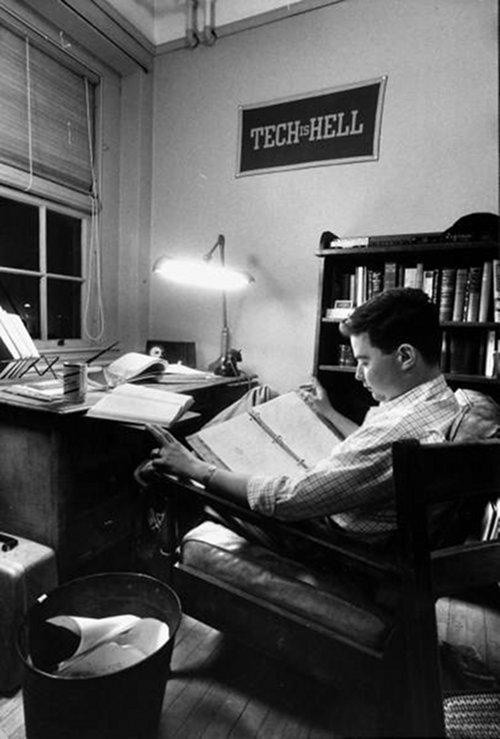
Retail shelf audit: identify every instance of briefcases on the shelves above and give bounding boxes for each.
[1,530,60,698]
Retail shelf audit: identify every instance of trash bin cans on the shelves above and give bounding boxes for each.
[15,572,182,739]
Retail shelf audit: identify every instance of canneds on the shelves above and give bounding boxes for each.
[63,362,87,404]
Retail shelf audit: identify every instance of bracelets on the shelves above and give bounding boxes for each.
[203,465,219,487]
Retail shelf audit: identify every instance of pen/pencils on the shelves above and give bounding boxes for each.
[85,340,120,363]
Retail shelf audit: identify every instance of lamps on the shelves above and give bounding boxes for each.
[153,234,256,377]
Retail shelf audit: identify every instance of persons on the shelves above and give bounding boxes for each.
[141,288,500,555]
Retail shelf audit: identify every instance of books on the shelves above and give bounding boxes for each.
[11,352,225,431]
[331,236,370,250]
[326,259,500,377]
[198,393,343,479]
[369,232,475,250]
[478,495,500,544]
[1,306,42,383]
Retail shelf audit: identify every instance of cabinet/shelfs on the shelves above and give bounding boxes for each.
[313,211,499,432]
